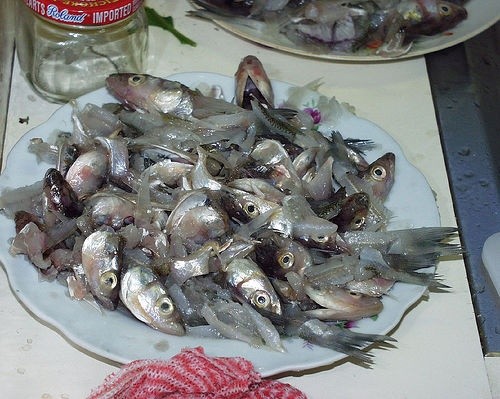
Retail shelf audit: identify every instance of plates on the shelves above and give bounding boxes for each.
[188,0,500,61]
[0,70,441,374]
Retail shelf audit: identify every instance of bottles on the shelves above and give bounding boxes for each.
[20,0,149,104]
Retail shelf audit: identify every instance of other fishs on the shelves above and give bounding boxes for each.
[185,0,469,58]
[9,55,468,369]
